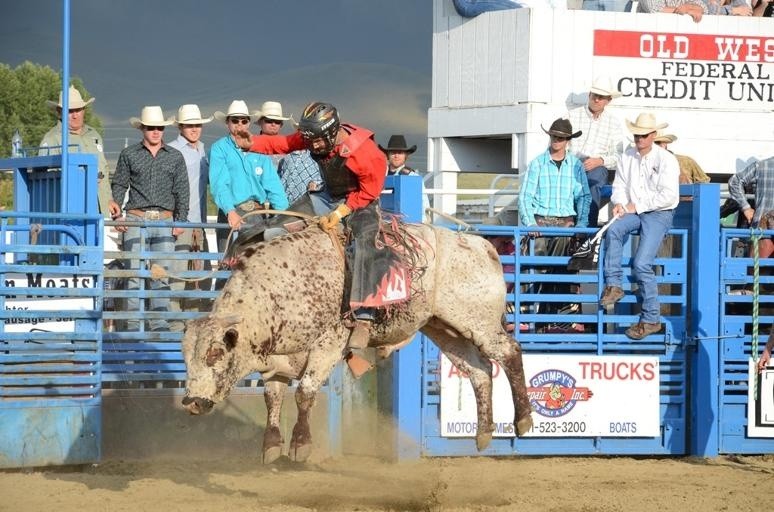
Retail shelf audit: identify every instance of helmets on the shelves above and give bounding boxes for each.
[299,101,340,159]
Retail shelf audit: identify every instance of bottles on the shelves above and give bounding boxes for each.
[10,127,23,159]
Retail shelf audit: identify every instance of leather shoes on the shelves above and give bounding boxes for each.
[626,317,664,341]
[599,285,626,312]
[348,319,373,352]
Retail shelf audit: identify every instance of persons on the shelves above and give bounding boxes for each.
[39,85,120,334]
[518,79,774,375]
[234,101,389,351]
[209,99,288,291]
[167,102,215,331]
[252,102,293,174]
[277,149,325,206]
[112,104,190,331]
[379,134,433,224]
[517,1,773,23]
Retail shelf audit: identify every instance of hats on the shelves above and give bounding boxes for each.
[539,117,583,140]
[588,77,623,99]
[623,112,679,144]
[172,103,215,126]
[376,133,419,158]
[45,87,97,110]
[249,100,291,123]
[128,98,176,129]
[214,99,259,127]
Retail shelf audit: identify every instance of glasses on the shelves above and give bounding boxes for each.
[263,117,282,125]
[228,118,250,126]
[144,124,165,131]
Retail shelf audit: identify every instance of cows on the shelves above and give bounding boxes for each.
[180,221,535,468]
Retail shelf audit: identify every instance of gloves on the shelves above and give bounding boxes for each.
[317,202,353,232]
